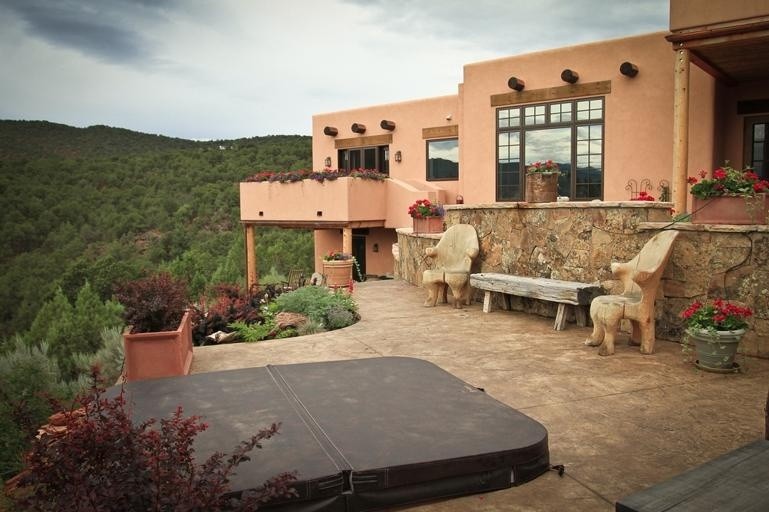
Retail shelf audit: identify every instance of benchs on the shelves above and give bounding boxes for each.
[470,272,599,330]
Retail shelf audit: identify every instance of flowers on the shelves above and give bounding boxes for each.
[527,159,558,173]
[680,298,750,370]
[323,248,352,260]
[685,159,769,225]
[408,200,444,222]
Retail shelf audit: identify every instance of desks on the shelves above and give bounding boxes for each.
[616,439,769,510]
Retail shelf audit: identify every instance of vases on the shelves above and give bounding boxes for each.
[323,260,353,288]
[686,329,745,369]
[525,173,559,203]
[413,217,442,233]
[692,193,768,224]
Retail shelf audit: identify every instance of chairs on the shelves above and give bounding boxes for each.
[584,228,680,357]
[422,223,480,309]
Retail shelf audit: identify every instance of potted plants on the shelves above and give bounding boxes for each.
[109,274,193,382]
